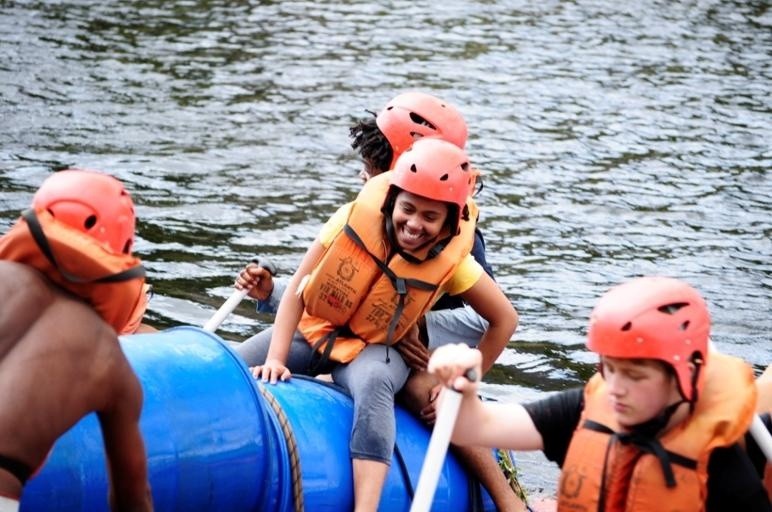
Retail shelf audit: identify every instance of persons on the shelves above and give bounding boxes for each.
[0,169,154,512]
[427,276,771,511]
[746,363,771,502]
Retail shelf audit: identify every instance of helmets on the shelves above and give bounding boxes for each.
[375,91,468,170]
[32,169,136,256]
[381,137,479,236]
[585,276,711,403]
